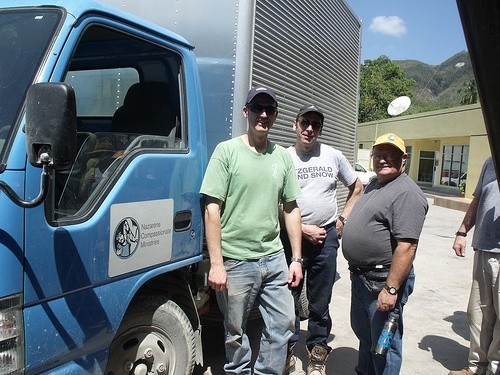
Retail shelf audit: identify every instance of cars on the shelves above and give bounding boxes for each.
[442,173,467,187]
[355,163,376,187]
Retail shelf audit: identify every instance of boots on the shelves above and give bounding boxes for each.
[283,342,306,375]
[304,343,333,375]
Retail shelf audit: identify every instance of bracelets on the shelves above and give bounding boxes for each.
[291,258,303,265]
[456,232,467,237]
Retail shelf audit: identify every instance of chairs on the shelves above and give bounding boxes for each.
[109,81,181,139]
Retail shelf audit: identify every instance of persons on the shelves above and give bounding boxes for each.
[341,133,429,375]
[448,157,500,375]
[200,85,303,375]
[276,105,364,375]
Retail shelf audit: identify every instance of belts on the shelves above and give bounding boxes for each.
[346,265,388,276]
[319,220,336,230]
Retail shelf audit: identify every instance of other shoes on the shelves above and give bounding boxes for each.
[447,367,477,375]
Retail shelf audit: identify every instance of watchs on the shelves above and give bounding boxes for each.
[384,284,399,295]
[338,216,348,226]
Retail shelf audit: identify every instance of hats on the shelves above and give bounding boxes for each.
[244,86,279,106]
[373,132,408,154]
[295,104,324,121]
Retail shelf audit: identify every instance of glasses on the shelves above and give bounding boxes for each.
[247,105,278,114]
[299,118,322,130]
[369,152,403,162]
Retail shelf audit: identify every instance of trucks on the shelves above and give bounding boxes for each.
[1,0,364,375]
